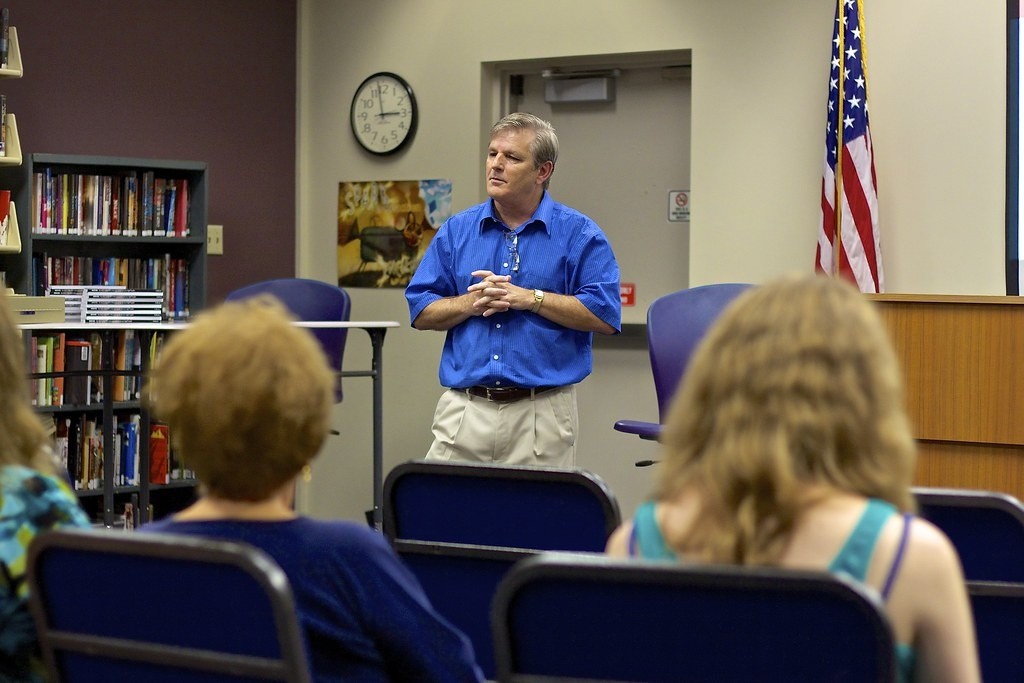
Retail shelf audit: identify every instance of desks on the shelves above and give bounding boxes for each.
[862,293,1024,504]
[18,321,399,530]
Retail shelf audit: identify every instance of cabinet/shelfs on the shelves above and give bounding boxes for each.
[0,22,23,255]
[23,153,209,526]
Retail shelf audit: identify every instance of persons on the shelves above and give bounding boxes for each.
[0,278,90,683]
[133,295,484,683]
[406,112,621,466]
[604,273,979,683]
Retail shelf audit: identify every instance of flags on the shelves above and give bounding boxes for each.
[814,0,885,293]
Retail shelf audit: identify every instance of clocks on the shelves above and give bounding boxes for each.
[350,72,419,157]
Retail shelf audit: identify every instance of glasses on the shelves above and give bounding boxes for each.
[506,229,520,274]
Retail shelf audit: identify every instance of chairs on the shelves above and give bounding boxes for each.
[910,486,1024,683]
[612,283,754,467]
[380,456,621,682]
[25,528,308,683]
[490,555,896,683]
[226,277,351,512]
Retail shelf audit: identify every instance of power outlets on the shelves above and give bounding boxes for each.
[207,225,224,256]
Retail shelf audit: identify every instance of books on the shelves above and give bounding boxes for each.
[0,7,9,69]
[17,329,196,532]
[33,252,191,323]
[33,163,193,238]
[0,95,7,157]
[0,190,10,246]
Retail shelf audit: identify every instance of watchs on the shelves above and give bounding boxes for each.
[531,289,544,313]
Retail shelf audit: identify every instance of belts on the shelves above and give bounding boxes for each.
[451,386,558,404]
